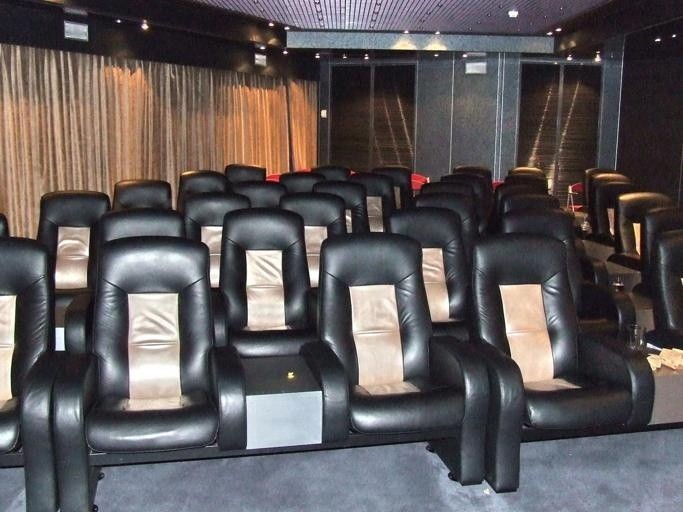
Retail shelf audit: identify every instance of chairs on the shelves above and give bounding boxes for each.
[279,172,326,195]
[301,233,491,507]
[646,230,683,348]
[589,174,629,217]
[208,208,318,355]
[510,168,545,174]
[312,166,351,185]
[586,182,636,246]
[13,192,112,374]
[313,182,368,234]
[635,206,683,291]
[504,174,551,183]
[228,182,288,209]
[178,171,228,210]
[410,194,474,248]
[439,176,486,206]
[348,176,396,235]
[181,193,252,285]
[280,194,346,292]
[453,170,492,193]
[495,185,545,202]
[112,180,172,213]
[97,211,185,239]
[419,183,476,196]
[585,168,616,208]
[0,214,8,236]
[484,207,638,318]
[470,235,656,493]
[372,169,413,213]
[224,163,264,186]
[500,196,561,219]
[54,236,246,508]
[394,209,472,340]
[0,235,55,507]
[608,193,673,265]
